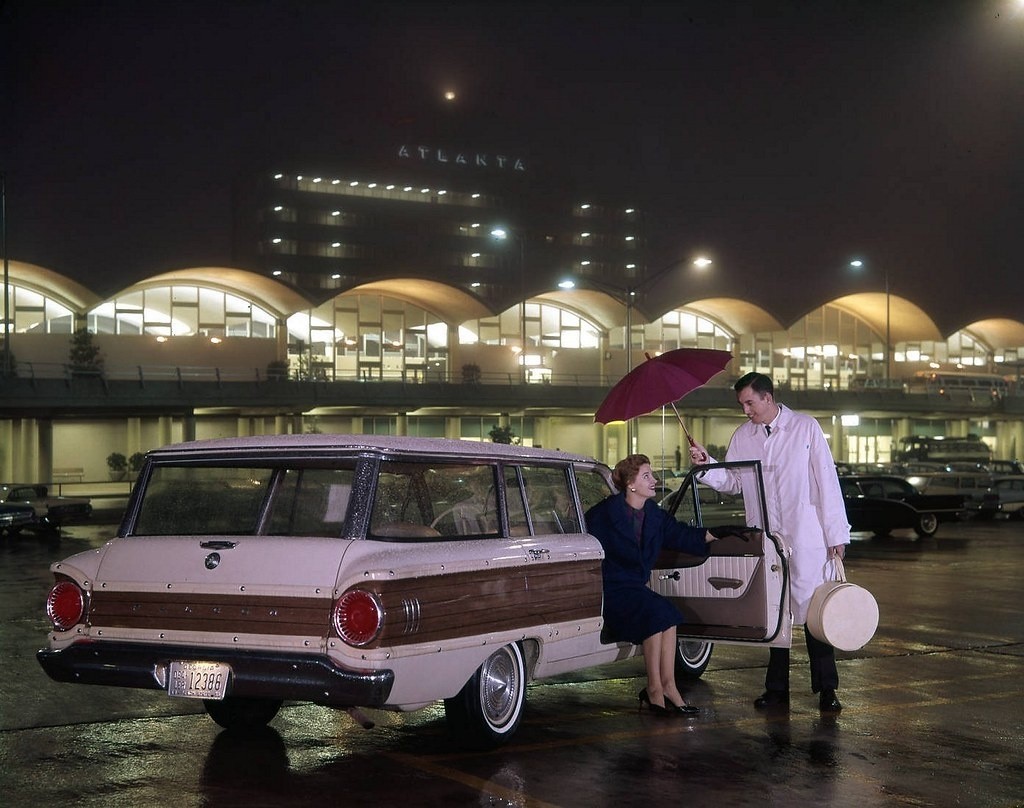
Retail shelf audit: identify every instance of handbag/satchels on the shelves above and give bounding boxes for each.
[807,553,879,651]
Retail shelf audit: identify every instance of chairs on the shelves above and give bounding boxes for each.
[375,522,562,557]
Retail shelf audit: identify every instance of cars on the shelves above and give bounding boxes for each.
[366,464,608,510]
[833,434,1024,540]
[35,434,794,753]
[650,466,762,542]
[0,481,93,539]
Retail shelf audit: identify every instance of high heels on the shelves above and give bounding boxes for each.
[663,694,700,715]
[638,688,669,718]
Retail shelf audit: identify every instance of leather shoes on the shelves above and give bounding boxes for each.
[820,690,842,711]
[754,691,789,708]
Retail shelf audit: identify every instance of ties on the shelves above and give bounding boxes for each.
[765,426,771,437]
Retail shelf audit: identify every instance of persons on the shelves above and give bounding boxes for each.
[689,372,852,711]
[585,454,761,717]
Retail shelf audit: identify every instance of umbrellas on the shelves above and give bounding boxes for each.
[595,348,732,462]
